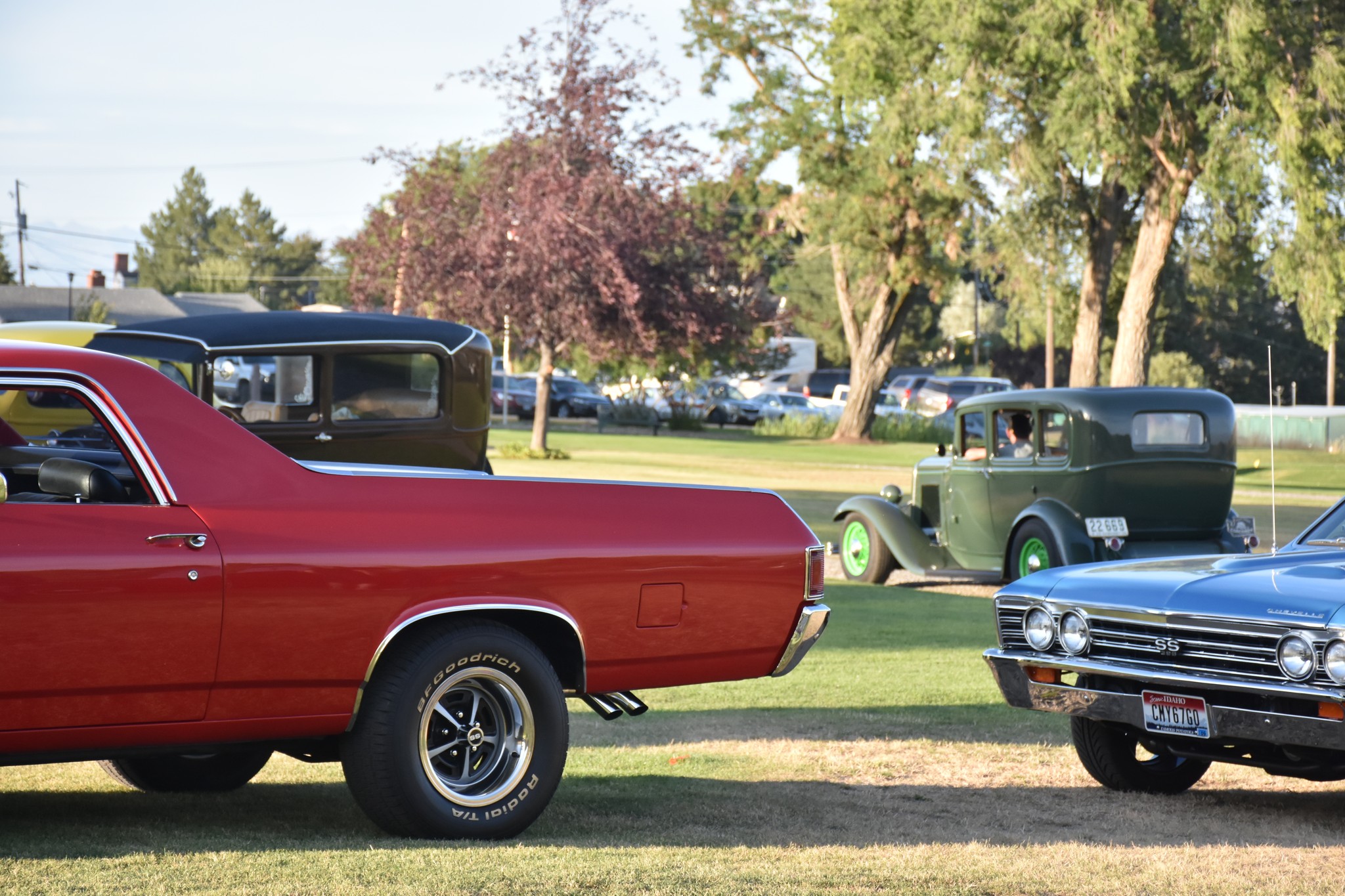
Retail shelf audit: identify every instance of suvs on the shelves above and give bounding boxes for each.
[916,376,1019,421]
[24,310,494,501]
[787,367,891,399]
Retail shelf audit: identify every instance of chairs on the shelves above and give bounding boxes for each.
[241,400,287,422]
[12,457,128,503]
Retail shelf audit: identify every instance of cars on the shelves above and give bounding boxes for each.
[984,489,1345,795]
[749,392,827,422]
[0,340,831,843]
[0,321,232,449]
[739,369,794,395]
[215,353,280,402]
[491,357,758,427]
[832,387,1261,590]
[887,374,939,413]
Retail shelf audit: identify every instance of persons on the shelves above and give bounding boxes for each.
[964,411,1051,461]
